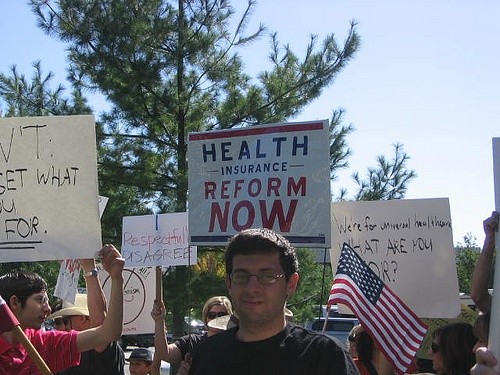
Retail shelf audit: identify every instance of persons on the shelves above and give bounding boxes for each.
[0,210,500,375]
[177,227,360,375]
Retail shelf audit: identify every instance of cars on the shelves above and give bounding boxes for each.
[312,304,357,318]
[460,289,494,324]
[184,316,204,335]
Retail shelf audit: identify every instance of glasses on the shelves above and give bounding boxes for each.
[208,312,227,320]
[61,317,69,325]
[18,296,48,304]
[432,341,448,353]
[230,271,285,284]
[52,319,62,326]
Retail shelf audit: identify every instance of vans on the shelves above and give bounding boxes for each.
[304,317,359,346]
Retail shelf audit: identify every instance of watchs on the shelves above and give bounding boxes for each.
[83,270,98,278]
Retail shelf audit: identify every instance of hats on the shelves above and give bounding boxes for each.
[125,349,152,364]
[45,294,107,320]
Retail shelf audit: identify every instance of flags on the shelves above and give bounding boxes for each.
[327,242,429,373]
